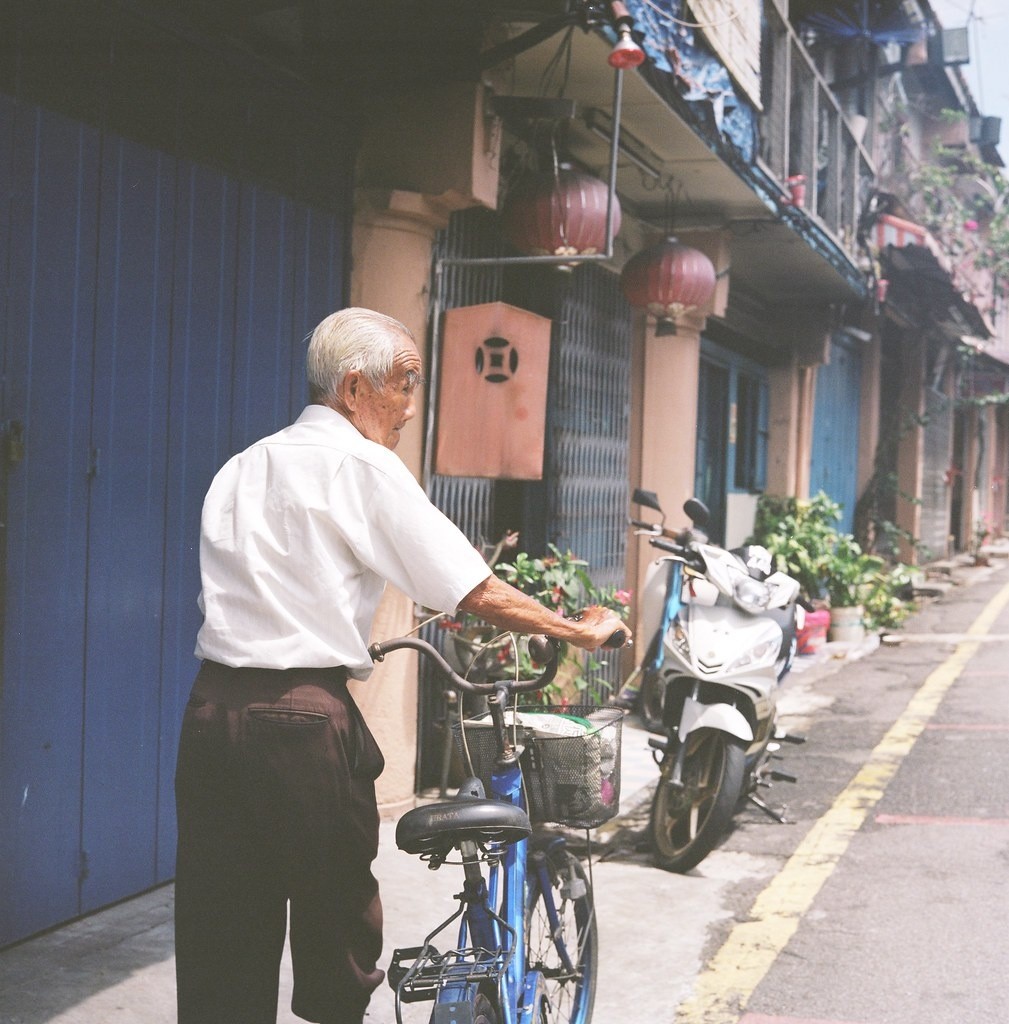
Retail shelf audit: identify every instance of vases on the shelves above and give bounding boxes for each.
[430,713,472,787]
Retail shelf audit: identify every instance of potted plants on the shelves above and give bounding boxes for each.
[746,489,885,653]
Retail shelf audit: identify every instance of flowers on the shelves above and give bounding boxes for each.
[436,543,631,712]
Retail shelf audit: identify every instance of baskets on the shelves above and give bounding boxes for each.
[452,703,624,828]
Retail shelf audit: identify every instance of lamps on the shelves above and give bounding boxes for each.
[606,0,645,70]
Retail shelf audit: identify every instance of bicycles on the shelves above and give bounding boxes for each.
[355,608,631,1024]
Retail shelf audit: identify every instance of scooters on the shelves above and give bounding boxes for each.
[618,484,817,871]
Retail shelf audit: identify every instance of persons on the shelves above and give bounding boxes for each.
[172,307,632,1024]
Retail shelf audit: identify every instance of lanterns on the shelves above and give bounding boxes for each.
[500,158,621,275]
[621,234,717,339]
